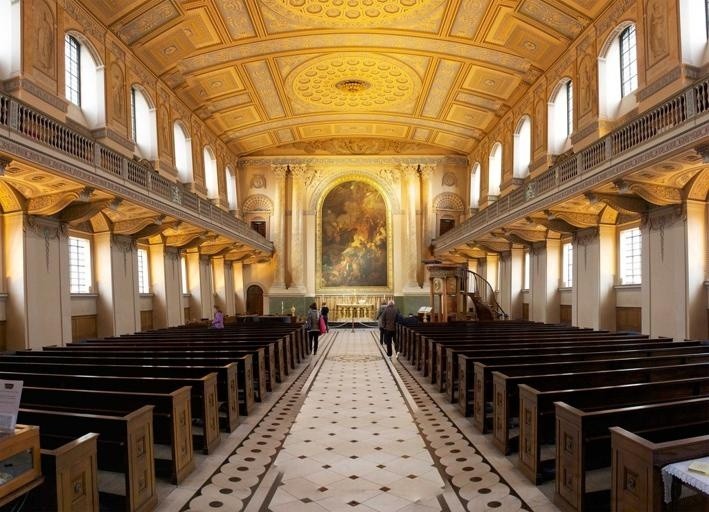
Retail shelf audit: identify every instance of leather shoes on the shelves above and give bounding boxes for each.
[314,351,318,355]
[310,350,312,354]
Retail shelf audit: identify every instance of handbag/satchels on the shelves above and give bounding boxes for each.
[319,315,327,335]
[305,315,313,330]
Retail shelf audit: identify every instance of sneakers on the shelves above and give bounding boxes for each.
[388,356,392,362]
[395,352,400,360]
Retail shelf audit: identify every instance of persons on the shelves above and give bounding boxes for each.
[321,302,330,333]
[306,303,321,355]
[211,305,224,328]
[380,300,400,360]
[376,299,387,345]
[406,313,418,324]
